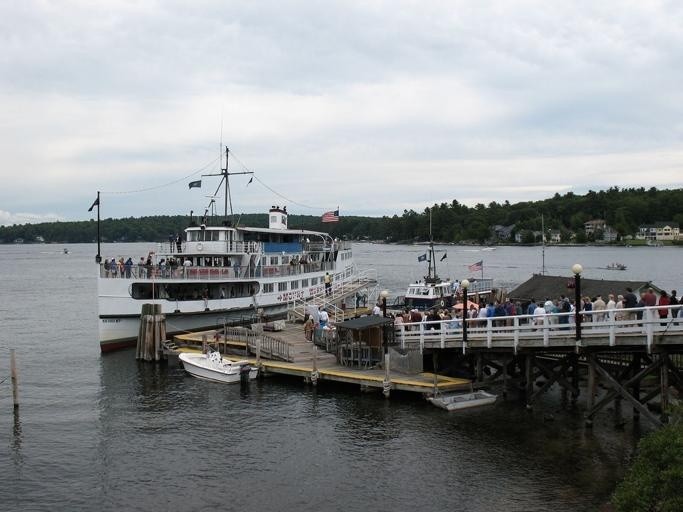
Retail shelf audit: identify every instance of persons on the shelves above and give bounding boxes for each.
[191,288,209,299]
[478,295,576,333]
[288,258,310,265]
[220,288,225,298]
[176,232,184,253]
[234,262,242,278]
[304,307,336,340]
[372,303,478,336]
[453,280,458,288]
[99,255,192,278]
[169,230,175,252]
[581,287,680,326]
[325,272,332,297]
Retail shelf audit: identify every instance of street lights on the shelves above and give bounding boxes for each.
[381,290,390,353]
[460,279,469,355]
[570,262,584,340]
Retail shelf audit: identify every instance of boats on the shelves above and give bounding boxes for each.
[177,349,259,385]
[402,206,497,308]
[425,388,498,412]
[95,148,376,357]
[482,247,496,252]
[607,264,627,270]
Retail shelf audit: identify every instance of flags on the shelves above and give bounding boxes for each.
[88,197,98,211]
[321,210,339,223]
[469,260,482,274]
[418,253,426,262]
[189,181,201,189]
[439,253,447,262]
